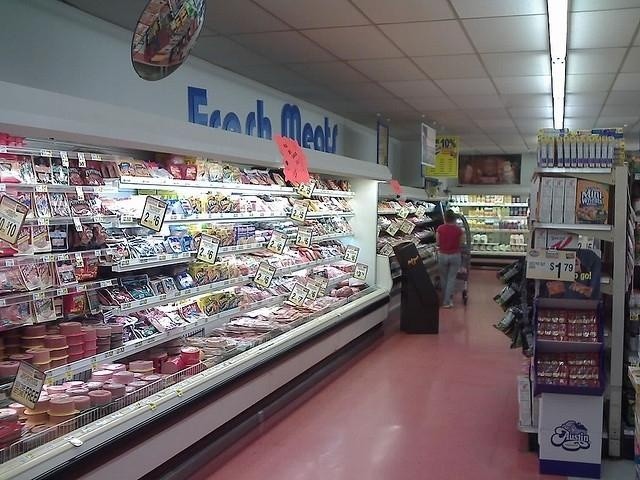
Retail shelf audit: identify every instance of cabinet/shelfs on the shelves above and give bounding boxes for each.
[444,194,530,254]
[0,146,377,476]
[517,161,640,479]
[378,194,441,279]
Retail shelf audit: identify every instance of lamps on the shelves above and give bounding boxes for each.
[545,0,570,132]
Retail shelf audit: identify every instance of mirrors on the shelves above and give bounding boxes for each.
[132,1,206,82]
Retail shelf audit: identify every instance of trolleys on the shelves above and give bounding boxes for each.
[435,243,472,305]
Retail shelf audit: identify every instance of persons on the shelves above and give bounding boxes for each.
[435,209,466,308]
